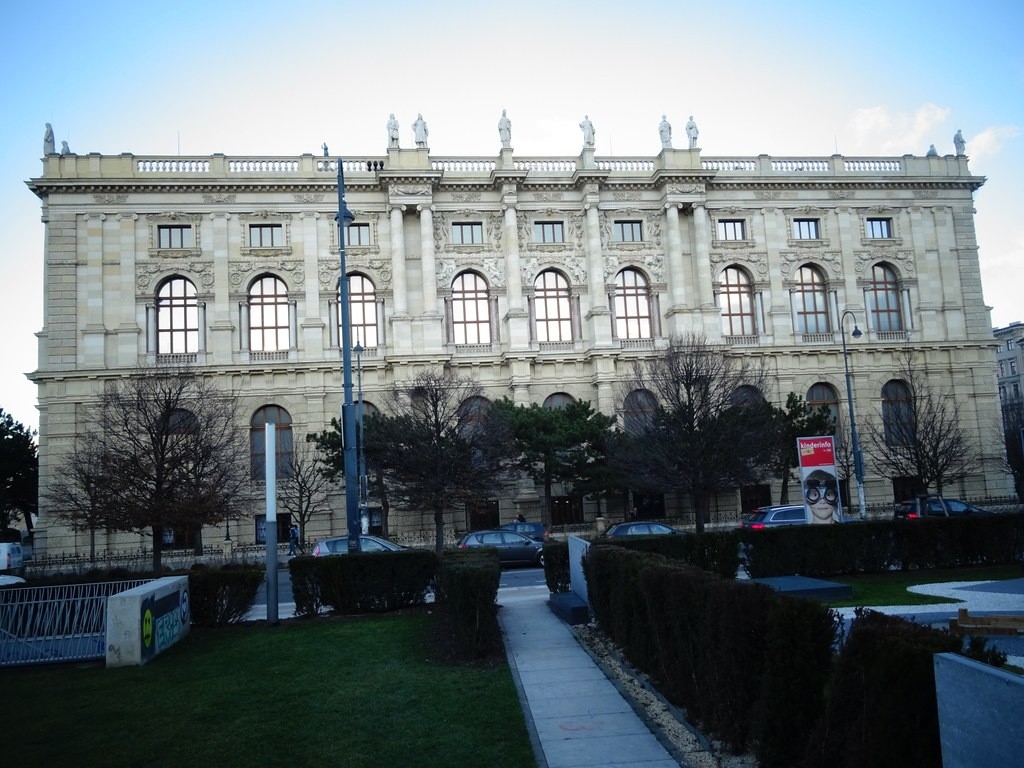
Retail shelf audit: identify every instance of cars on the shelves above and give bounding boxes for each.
[494,522,549,542]
[600,519,688,541]
[893,496,996,520]
[311,534,411,557]
[456,529,546,568]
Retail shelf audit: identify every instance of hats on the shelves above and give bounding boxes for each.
[518,513,522,516]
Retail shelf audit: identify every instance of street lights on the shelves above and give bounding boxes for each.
[334,157,363,556]
[841,311,867,520]
[360,338,369,535]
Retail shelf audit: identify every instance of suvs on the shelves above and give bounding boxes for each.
[738,505,863,530]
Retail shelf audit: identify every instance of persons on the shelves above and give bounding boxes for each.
[512,510,526,523]
[802,469,840,525]
[285,523,306,556]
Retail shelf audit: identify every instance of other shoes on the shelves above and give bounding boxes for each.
[287,554,291,555]
[293,554,296,555]
[300,552,304,555]
